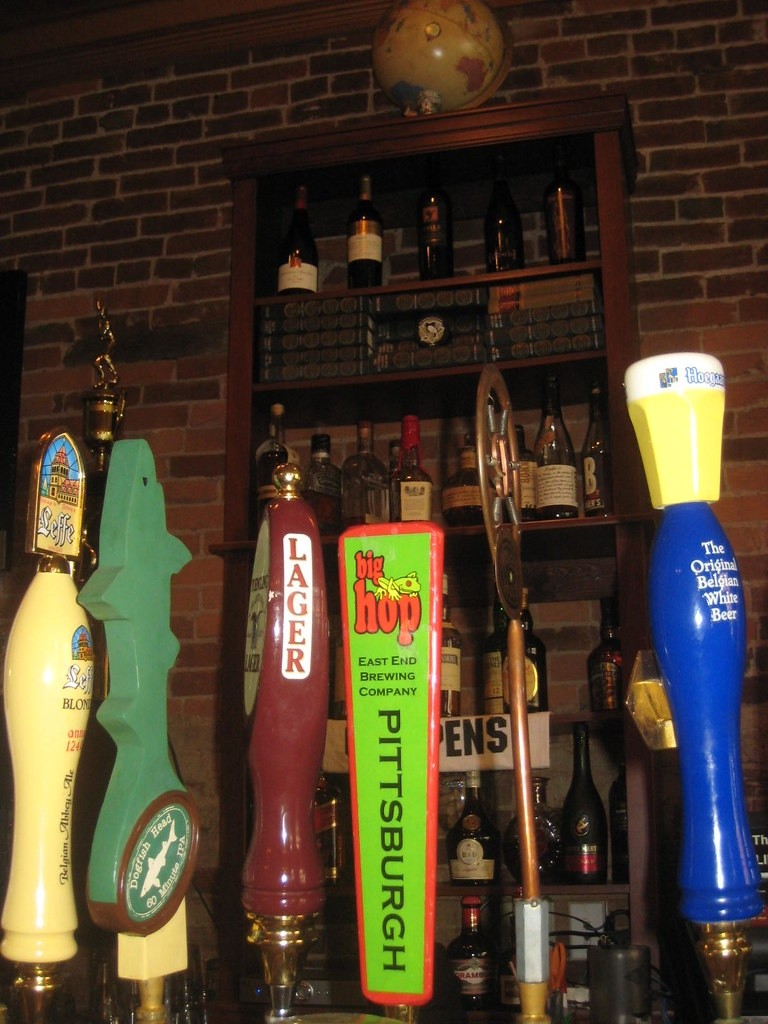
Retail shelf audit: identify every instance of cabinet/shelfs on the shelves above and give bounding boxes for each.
[208,89,645,1005]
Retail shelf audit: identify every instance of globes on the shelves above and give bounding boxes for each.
[370,0,514,116]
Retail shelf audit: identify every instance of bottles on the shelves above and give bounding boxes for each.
[542,157,588,267]
[274,185,318,297]
[444,722,631,887]
[311,771,346,885]
[586,596,626,709]
[479,580,550,715]
[482,173,526,274]
[345,172,383,291]
[247,371,605,540]
[417,166,454,282]
[437,573,463,717]
[443,896,522,1024]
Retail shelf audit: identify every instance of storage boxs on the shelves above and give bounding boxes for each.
[256,272,608,383]
